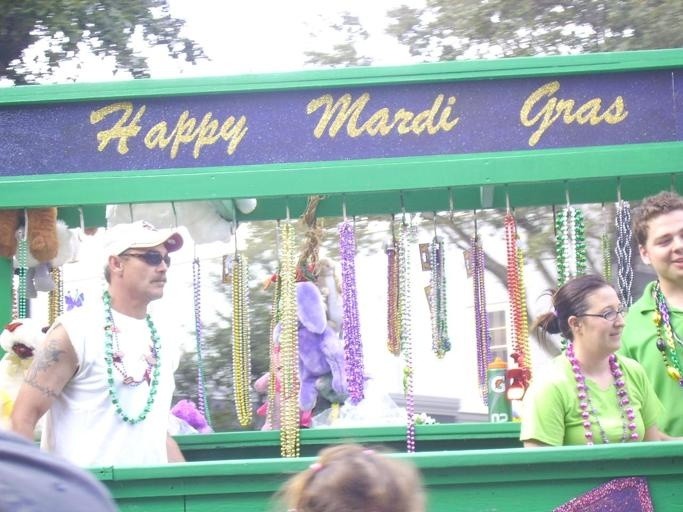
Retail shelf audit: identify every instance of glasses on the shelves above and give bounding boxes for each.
[567,303,627,322]
[115,248,171,266]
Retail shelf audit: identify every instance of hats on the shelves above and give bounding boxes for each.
[102,220,184,267]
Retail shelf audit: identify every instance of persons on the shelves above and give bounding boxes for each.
[272,442,428,511]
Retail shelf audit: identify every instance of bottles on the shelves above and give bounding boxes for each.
[487,356,512,421]
